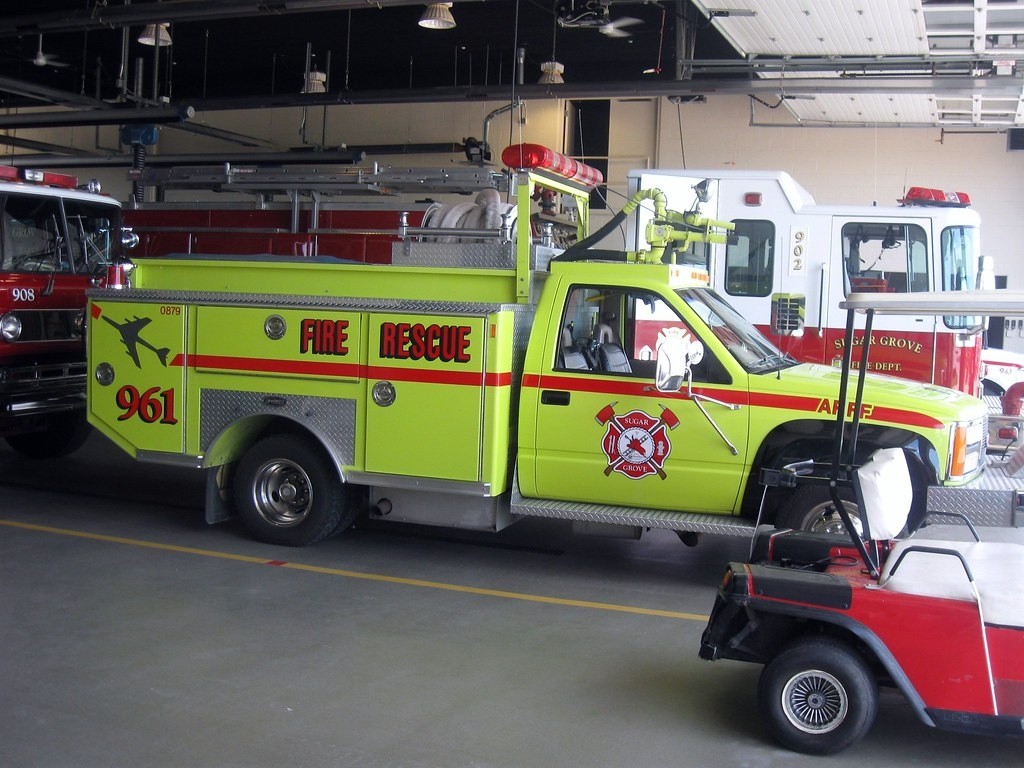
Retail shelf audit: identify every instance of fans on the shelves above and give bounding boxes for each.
[33,34,68,67]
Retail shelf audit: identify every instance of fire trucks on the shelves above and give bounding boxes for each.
[0,166,141,462]
[76,141,1020,563]
[0,147,1022,452]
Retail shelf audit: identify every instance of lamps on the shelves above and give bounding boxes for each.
[418,2,456,29]
[138,23,172,46]
[537,61,565,84]
[301,71,326,93]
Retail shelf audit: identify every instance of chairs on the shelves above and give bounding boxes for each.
[594,324,632,373]
[855,448,1024,631]
[557,327,590,370]
